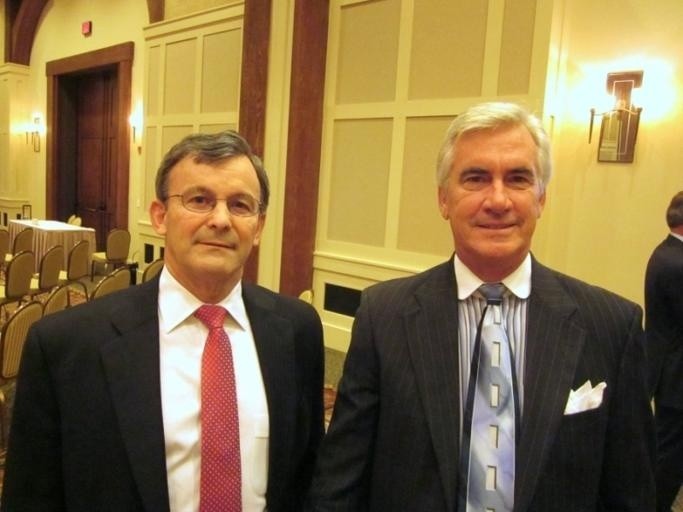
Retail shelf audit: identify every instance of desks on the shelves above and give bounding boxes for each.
[7,219,95,272]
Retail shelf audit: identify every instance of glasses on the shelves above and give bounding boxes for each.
[163,188,262,217]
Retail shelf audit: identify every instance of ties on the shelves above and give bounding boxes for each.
[464,282,516,512]
[193,304,242,512]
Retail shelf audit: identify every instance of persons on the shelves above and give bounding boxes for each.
[301,101,658,511]
[1,128,326,512]
[642,191,683,511]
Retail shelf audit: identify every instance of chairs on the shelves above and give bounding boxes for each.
[0,227,166,386]
